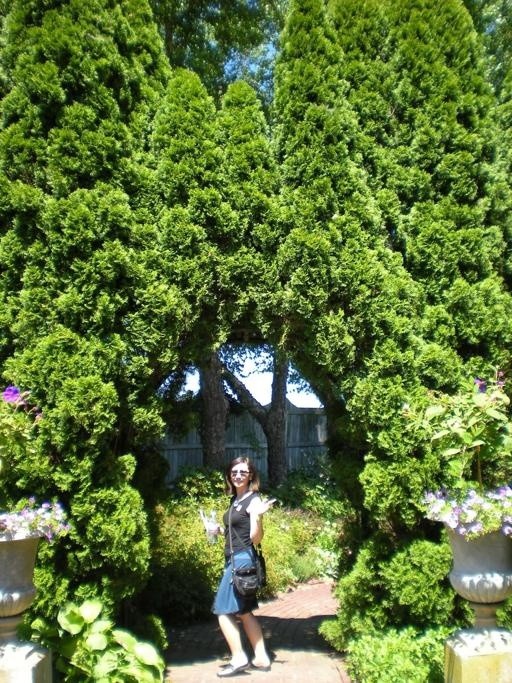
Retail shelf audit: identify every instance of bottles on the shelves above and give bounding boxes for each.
[207,510,219,543]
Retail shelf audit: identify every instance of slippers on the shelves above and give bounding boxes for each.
[217,661,270,677]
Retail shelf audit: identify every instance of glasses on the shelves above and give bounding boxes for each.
[232,470,252,475]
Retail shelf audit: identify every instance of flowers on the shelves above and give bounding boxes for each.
[0,496,72,547]
[420,484,512,540]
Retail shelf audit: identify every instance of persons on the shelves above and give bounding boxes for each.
[198,455,274,677]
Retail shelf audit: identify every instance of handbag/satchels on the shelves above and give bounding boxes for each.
[232,556,266,600]
